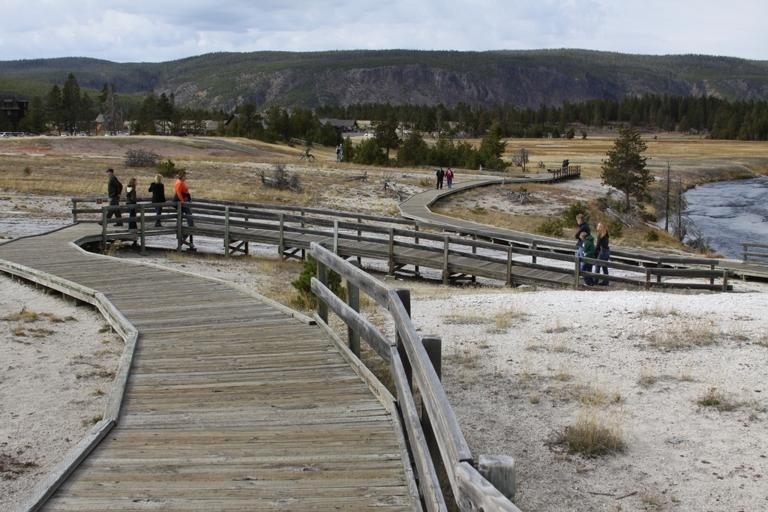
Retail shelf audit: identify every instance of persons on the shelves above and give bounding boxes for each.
[578,230,595,286]
[146,174,166,228]
[125,177,137,229]
[99,170,123,227]
[595,221,610,285]
[171,169,195,228]
[436,166,444,190]
[574,210,591,273]
[444,167,453,189]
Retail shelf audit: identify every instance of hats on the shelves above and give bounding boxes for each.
[106,169,113,173]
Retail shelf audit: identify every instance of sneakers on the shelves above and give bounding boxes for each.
[583,280,610,287]
[98,220,123,226]
